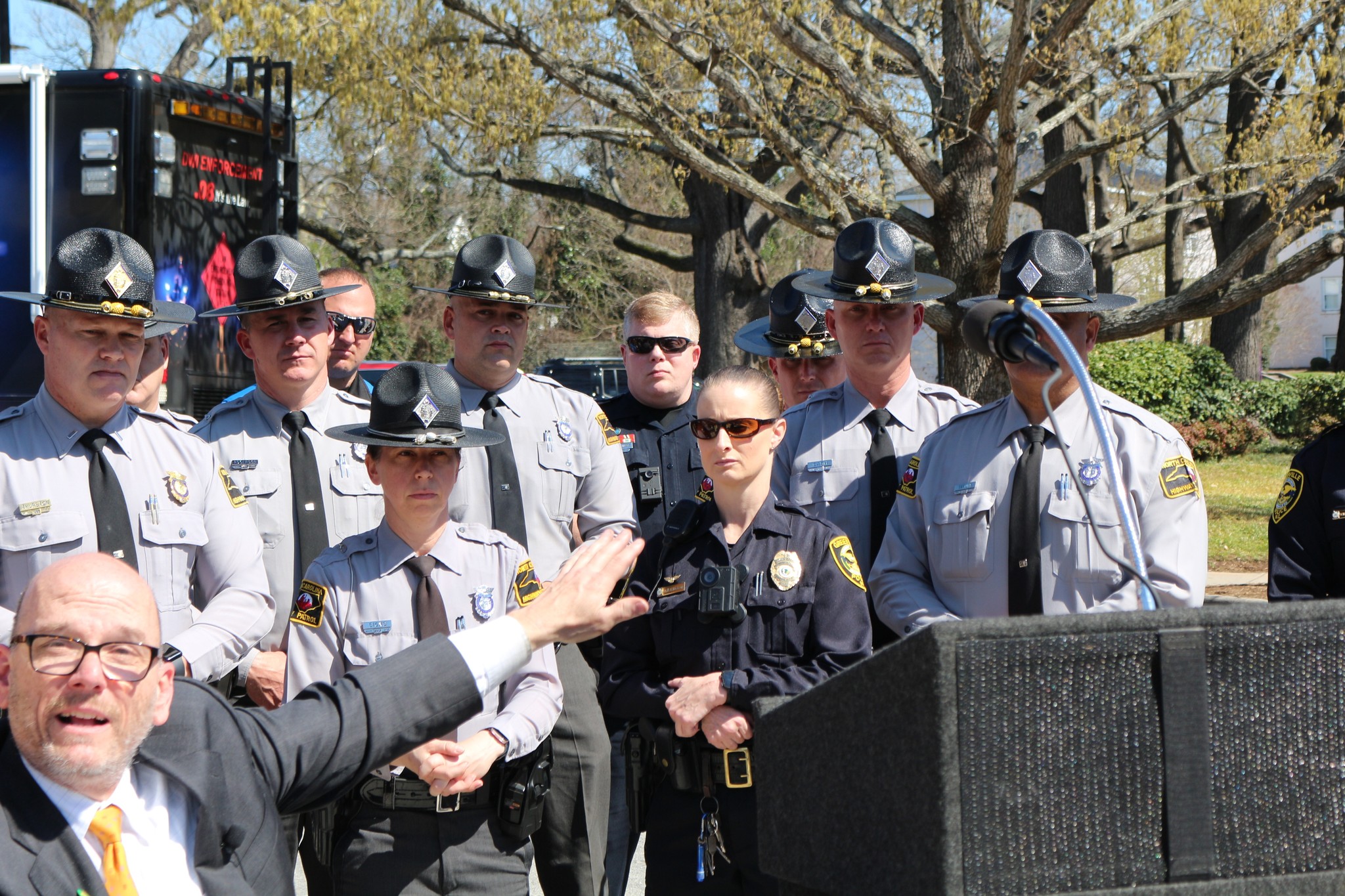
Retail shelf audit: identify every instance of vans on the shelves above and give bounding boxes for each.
[532,357,631,403]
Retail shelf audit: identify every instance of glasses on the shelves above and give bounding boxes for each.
[689,418,779,440]
[324,310,378,335]
[10,634,160,682]
[626,336,699,354]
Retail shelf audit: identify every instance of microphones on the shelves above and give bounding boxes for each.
[961,301,1058,372]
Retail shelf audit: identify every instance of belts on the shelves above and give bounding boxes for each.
[358,775,501,812]
[712,745,757,789]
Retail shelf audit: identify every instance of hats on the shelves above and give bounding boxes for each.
[140,281,195,340]
[958,230,1136,314]
[199,233,363,320]
[411,234,568,308]
[323,363,505,448]
[791,218,957,305]
[0,229,197,327]
[733,269,844,358]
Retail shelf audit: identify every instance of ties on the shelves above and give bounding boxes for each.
[478,392,528,555]
[865,407,897,650]
[78,427,137,572]
[282,409,330,578]
[404,553,458,744]
[87,805,137,896]
[1008,425,1045,615]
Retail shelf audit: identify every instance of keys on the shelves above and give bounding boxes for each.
[697,812,730,877]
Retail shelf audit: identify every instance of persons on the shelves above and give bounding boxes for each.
[769,219,981,653]
[125,286,200,434]
[407,235,643,896]
[1264,423,1345,602]
[733,269,847,409]
[0,228,277,686]
[867,231,1209,641]
[600,364,873,896]
[1,529,649,896]
[577,291,715,896]
[187,234,389,714]
[284,362,563,896]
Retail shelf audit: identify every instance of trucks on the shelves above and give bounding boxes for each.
[0,0,300,425]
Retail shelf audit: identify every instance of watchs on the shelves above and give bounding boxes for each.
[720,670,735,706]
[488,727,509,760]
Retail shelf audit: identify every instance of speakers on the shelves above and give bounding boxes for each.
[746,594,1345,896]
